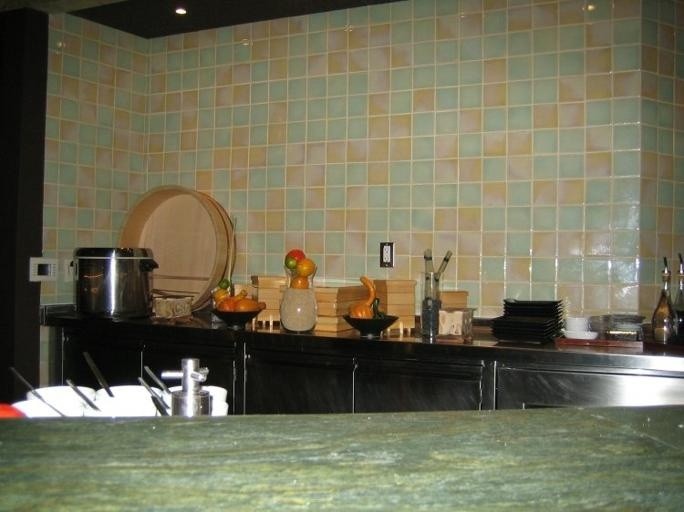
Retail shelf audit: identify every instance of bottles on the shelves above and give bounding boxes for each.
[651,253,684,346]
[419,246,452,335]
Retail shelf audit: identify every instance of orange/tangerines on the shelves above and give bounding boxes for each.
[218,279,229,289]
[285,256,297,270]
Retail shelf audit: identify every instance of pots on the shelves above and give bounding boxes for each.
[68,246,159,323]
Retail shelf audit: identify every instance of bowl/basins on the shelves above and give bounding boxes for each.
[211,306,264,330]
[341,312,399,337]
[0,379,229,421]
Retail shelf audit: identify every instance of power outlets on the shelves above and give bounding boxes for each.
[379,241,394,268]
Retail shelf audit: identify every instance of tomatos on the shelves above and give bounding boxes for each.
[288,249,304,262]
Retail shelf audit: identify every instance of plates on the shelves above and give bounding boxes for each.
[566,313,651,341]
[488,297,568,346]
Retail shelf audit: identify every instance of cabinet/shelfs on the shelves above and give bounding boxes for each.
[62,326,234,415]
[495,361,683,411]
[244,342,481,416]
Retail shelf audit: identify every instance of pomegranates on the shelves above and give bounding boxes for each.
[218,298,265,312]
